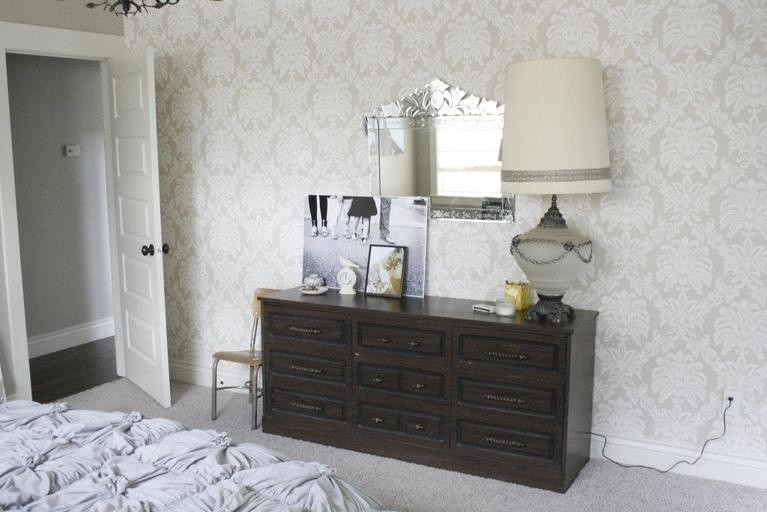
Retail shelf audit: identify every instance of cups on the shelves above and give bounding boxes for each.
[496,296,516,317]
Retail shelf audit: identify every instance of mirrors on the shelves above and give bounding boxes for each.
[360,79,515,224]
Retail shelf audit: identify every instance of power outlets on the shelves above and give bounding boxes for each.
[722,389,740,416]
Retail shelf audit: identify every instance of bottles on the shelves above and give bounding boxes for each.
[304,274,324,287]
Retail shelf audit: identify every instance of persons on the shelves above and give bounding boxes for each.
[347,197,377,245]
[307,196,328,237]
[379,197,396,244]
[330,196,352,240]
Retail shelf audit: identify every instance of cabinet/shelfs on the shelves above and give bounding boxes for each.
[256,288,599,493]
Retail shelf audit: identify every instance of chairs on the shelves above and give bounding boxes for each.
[213,288,281,428]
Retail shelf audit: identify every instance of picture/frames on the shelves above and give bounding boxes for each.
[364,245,407,299]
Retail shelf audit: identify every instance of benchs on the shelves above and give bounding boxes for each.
[0,398,384,512]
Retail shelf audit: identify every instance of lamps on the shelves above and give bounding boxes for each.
[502,58,609,325]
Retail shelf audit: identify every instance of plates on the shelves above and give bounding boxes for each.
[299,287,328,295]
